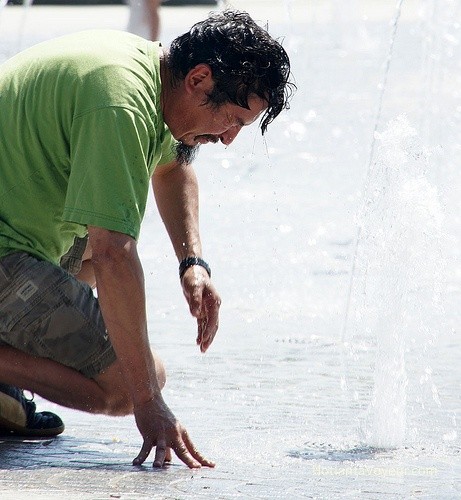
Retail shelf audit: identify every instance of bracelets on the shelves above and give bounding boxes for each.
[178,257,211,281]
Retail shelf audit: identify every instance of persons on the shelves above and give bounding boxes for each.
[0,9,296,470]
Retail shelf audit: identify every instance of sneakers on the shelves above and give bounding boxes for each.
[0,382,64,437]
